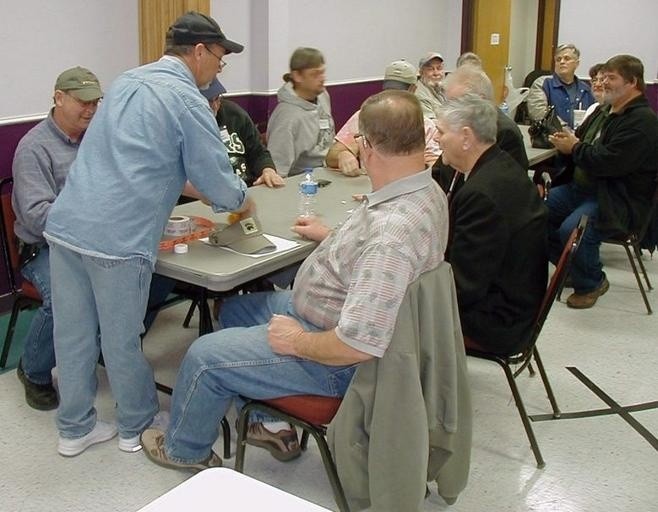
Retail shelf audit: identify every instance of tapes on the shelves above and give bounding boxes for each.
[167,216,191,232]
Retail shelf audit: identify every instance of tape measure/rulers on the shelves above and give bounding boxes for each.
[159,215,216,248]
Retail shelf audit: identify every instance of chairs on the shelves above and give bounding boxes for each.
[230,258,451,512]
[601,199,656,319]
[460,214,590,471]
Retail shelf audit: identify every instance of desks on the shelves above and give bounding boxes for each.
[133,464,333,512]
[147,124,559,460]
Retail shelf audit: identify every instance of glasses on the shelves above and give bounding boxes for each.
[200,43,227,69]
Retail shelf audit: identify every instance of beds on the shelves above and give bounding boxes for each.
[0,176,46,374]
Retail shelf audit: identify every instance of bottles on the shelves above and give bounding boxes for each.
[297,168,318,218]
[498,101,508,115]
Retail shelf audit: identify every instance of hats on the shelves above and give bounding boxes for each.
[206,214,278,256]
[382,57,418,87]
[417,52,444,69]
[52,64,105,104]
[163,9,245,55]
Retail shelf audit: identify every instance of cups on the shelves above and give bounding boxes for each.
[573,109,586,130]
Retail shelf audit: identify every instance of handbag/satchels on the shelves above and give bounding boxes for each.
[526,103,566,150]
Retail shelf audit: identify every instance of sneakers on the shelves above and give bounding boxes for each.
[56,416,122,460]
[563,268,610,310]
[118,406,173,452]
[16,354,61,413]
[233,416,306,464]
[139,426,224,475]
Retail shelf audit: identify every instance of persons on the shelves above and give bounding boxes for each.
[539,53,657,310]
[430,91,553,362]
[431,65,531,196]
[7,64,176,414]
[138,85,454,477]
[39,9,262,459]
[320,59,443,178]
[518,42,597,182]
[262,44,337,180]
[177,74,288,210]
[576,60,607,127]
[412,51,448,125]
[454,50,484,76]
